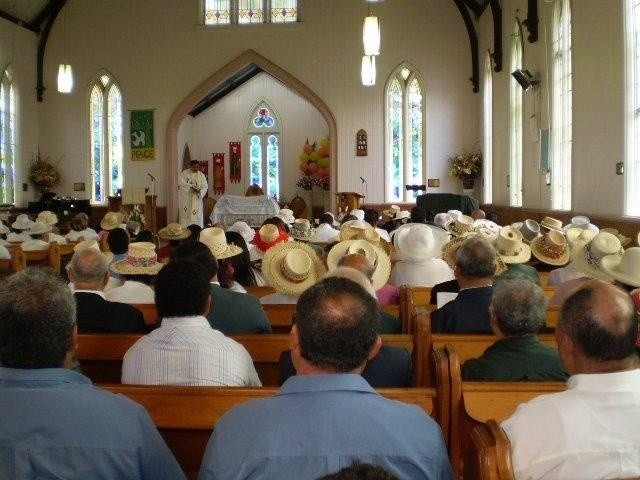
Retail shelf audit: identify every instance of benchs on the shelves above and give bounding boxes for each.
[0,222,640,480]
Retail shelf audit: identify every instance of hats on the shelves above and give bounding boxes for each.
[488,225,531,265]
[383,204,401,217]
[600,228,631,246]
[568,228,594,249]
[511,219,543,246]
[261,241,326,294]
[564,215,599,237]
[0,211,124,235]
[276,209,295,224]
[110,242,166,275]
[327,239,391,292]
[158,223,191,241]
[314,212,340,226]
[253,223,289,251]
[392,211,411,220]
[573,232,625,284]
[392,223,452,261]
[289,218,316,241]
[602,246,640,288]
[539,216,566,237]
[434,209,501,268]
[530,229,570,265]
[339,220,391,256]
[66,241,113,272]
[227,221,256,243]
[200,228,243,261]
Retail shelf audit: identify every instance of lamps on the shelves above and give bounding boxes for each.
[363,0,381,56]
[360,52,378,88]
[512,68,540,91]
[57,0,74,93]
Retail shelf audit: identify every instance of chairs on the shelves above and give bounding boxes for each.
[204,185,307,227]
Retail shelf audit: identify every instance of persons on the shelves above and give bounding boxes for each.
[178,160,208,229]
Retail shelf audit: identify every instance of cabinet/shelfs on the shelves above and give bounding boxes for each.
[27,199,92,218]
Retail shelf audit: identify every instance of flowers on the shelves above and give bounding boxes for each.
[314,177,329,191]
[446,134,484,177]
[295,177,313,190]
[32,158,61,191]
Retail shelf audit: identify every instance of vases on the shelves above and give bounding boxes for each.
[463,178,474,189]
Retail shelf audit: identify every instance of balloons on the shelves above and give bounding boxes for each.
[300,139,331,177]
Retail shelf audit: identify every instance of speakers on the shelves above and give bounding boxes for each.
[510,68,534,92]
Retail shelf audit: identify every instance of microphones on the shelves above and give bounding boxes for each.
[360,176,367,183]
[148,173,155,181]
